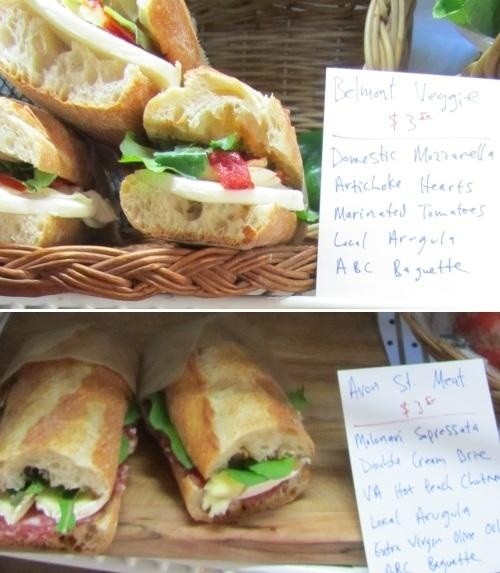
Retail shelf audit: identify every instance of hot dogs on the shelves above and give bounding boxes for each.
[0,0,308,249]
[2,307,317,558]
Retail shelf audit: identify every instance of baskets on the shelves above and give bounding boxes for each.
[0,0,415,298]
